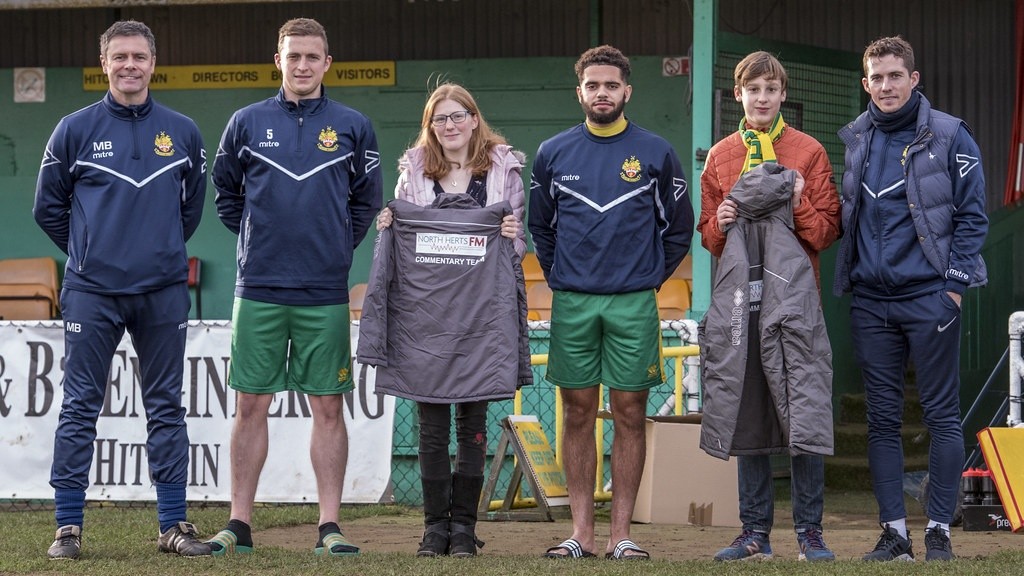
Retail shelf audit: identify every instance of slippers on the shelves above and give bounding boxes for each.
[542,538,596,558]
[606,539,650,560]
[314,533,361,556]
[203,529,252,558]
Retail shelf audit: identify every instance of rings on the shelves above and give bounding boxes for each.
[378,219,382,223]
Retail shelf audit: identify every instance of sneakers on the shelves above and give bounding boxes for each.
[48,524,82,562]
[861,521,915,565]
[158,521,212,558]
[713,524,774,561]
[797,530,834,563]
[924,524,952,563]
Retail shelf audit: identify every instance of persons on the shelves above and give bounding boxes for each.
[696,52,844,560]
[828,37,986,565]
[32,18,214,561]
[373,79,527,560]
[201,18,384,553]
[527,46,694,559]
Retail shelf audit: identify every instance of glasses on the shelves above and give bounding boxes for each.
[430,110,469,126]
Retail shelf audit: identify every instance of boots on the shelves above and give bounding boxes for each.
[450,472,485,558]
[416,475,450,557]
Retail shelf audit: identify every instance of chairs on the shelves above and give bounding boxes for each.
[187,256,202,319]
[0,258,60,320]
[523,253,556,321]
[656,255,692,321]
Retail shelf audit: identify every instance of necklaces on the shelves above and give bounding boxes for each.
[446,169,466,186]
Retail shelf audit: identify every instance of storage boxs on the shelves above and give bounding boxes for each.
[596,410,743,527]
